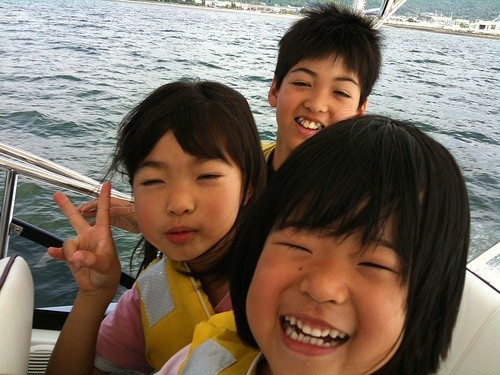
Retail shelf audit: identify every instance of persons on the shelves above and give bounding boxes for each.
[151,115,470,375]
[46,72,281,374]
[76,0,388,235]
